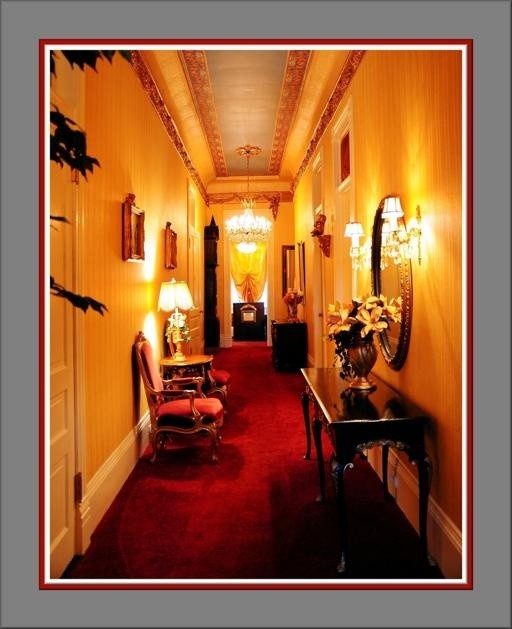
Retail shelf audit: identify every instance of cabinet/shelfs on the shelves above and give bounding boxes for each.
[233,303,266,339]
[270,319,308,373]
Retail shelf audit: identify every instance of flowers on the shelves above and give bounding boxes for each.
[325,294,389,377]
[284,290,303,304]
[390,297,401,323]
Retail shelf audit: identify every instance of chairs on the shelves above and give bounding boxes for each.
[134,331,226,463]
[166,323,231,415]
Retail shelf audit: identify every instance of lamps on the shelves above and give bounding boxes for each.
[381,196,421,264]
[223,146,272,254]
[345,222,372,259]
[380,223,400,268]
[156,278,194,362]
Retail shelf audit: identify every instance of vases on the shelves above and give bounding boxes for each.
[347,340,378,393]
[288,302,298,322]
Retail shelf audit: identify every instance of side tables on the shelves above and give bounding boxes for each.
[160,355,213,388]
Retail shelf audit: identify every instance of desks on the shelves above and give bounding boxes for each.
[299,366,432,572]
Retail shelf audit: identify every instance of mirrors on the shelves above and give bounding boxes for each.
[371,195,413,371]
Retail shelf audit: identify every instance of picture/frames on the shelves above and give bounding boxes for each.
[165,222,178,269]
[282,245,295,298]
[123,193,145,262]
